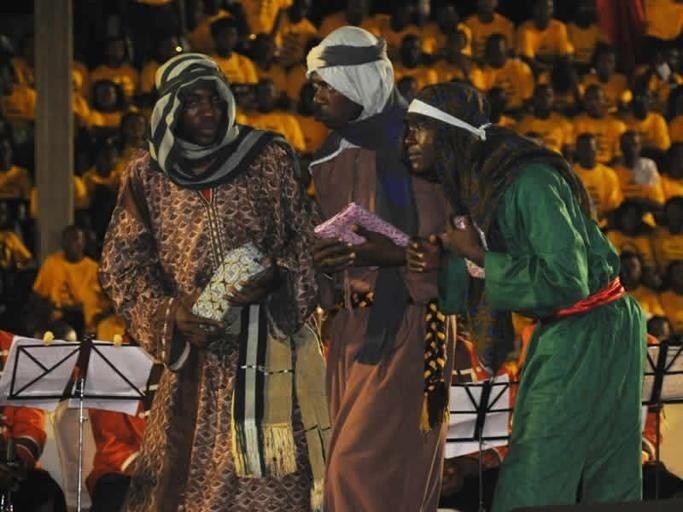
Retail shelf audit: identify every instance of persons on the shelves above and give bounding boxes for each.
[0,0,683,512]
